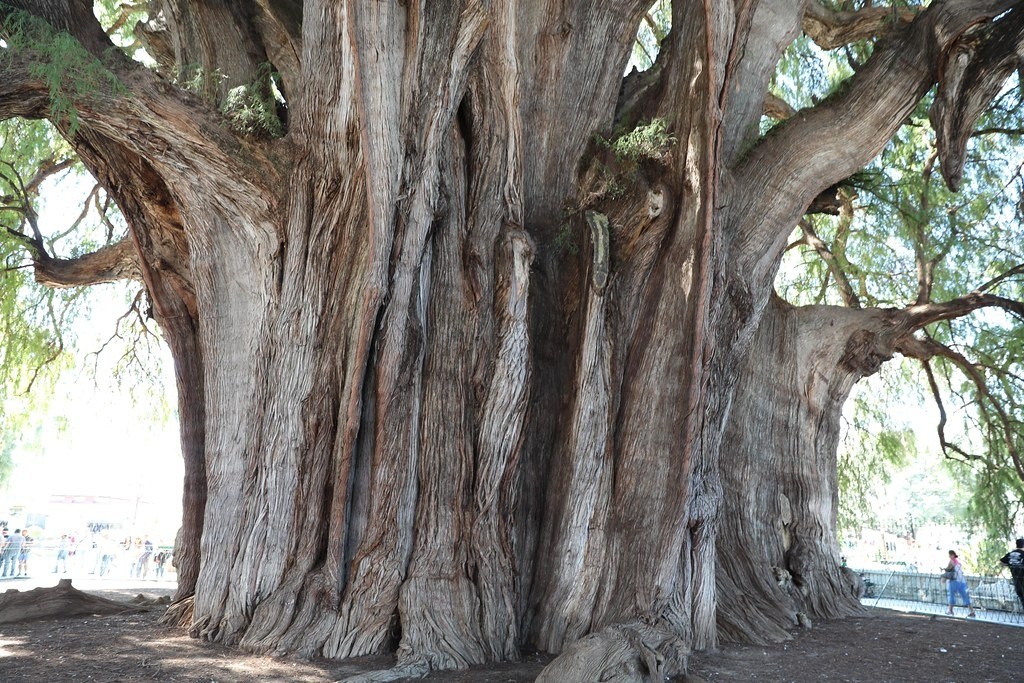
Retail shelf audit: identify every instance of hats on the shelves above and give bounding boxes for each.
[1016,539,1024,548]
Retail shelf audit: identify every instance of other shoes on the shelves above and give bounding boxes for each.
[17,573,27,577]
[1,574,13,577]
[946,612,954,615]
[968,612,975,617]
[51,569,68,573]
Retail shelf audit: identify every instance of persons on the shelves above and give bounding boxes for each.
[1000,539,1024,610]
[51,531,173,578]
[939,550,976,617]
[839,556,847,566]
[0,528,34,577]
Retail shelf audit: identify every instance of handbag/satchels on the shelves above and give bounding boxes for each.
[941,570,958,580]
